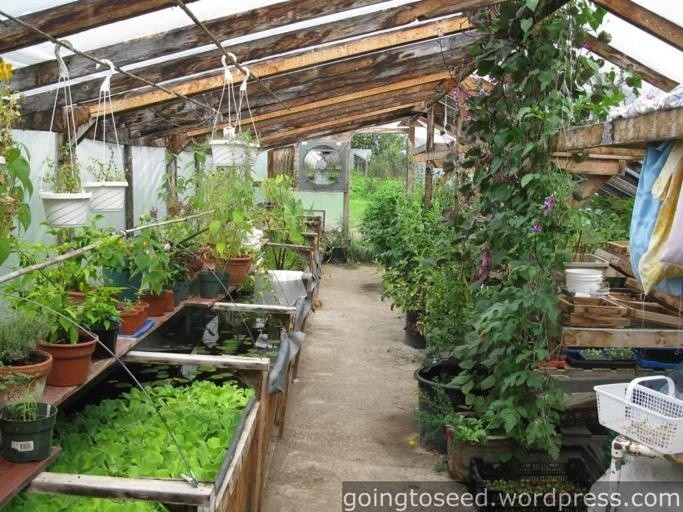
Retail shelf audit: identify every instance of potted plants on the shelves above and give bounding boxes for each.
[211,181,252,290]
[445,197,578,485]
[164,218,191,305]
[72,231,119,359]
[1,225,127,387]
[207,51,246,168]
[0,373,58,463]
[412,236,493,454]
[133,238,175,318]
[235,67,260,165]
[0,309,53,404]
[37,40,90,229]
[82,58,127,213]
[406,217,432,348]
[118,299,149,335]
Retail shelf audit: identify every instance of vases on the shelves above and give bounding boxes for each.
[200,270,231,298]
